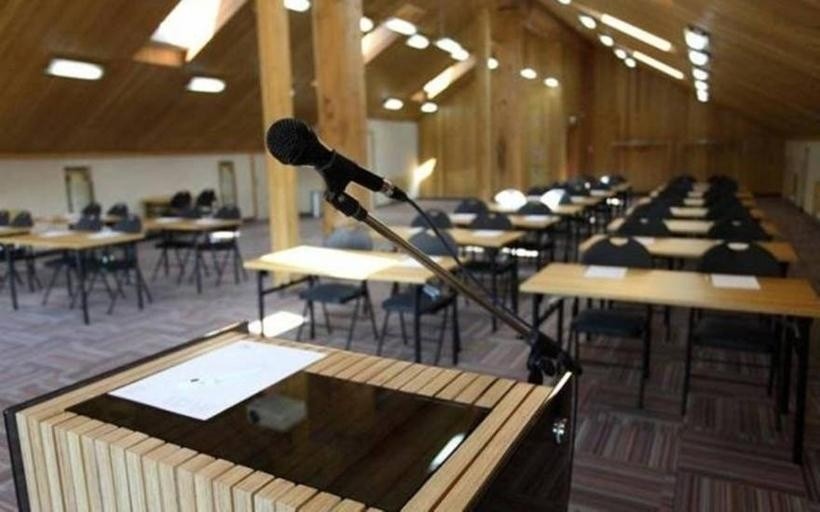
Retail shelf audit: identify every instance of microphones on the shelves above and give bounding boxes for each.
[268,119,407,203]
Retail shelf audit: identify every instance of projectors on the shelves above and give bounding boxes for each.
[247,394,307,432]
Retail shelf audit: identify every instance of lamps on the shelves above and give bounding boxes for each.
[679,24,713,106]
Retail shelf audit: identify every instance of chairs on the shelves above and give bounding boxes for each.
[1,188,248,324]
